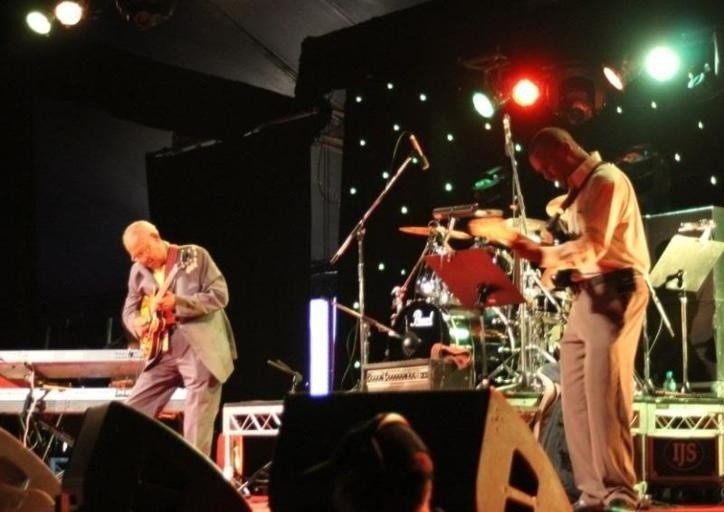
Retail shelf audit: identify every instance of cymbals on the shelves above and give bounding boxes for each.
[503,217,546,232]
[546,195,570,220]
[399,227,468,240]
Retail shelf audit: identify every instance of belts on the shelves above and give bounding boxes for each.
[569,266,634,295]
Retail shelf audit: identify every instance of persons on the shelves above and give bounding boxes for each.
[333,409,438,511]
[509,125,652,511]
[120,218,240,465]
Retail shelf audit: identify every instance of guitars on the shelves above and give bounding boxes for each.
[138,247,195,360]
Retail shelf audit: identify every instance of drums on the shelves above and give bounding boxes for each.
[388,302,518,386]
[534,296,571,323]
[469,243,513,277]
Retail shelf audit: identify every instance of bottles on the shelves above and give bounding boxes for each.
[663,370,676,392]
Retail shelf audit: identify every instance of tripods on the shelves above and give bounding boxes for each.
[476,256,567,390]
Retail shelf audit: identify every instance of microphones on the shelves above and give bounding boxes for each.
[409,134,429,171]
[504,113,512,156]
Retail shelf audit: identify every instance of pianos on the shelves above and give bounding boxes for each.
[0,350,186,413]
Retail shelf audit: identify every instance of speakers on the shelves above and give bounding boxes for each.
[62,401,252,512]
[269,388,575,512]
[645,205,724,396]
